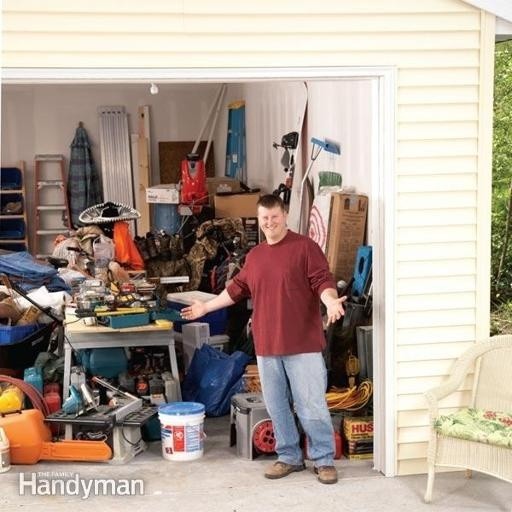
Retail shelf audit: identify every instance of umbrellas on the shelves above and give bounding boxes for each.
[62,122,102,231]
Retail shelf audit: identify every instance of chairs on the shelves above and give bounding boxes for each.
[423,334,512,504]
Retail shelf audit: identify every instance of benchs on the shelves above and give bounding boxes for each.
[174,332,230,374]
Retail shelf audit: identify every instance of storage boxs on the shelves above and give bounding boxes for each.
[0,326,34,344]
[324,192,369,283]
[0,323,49,371]
[145,183,180,205]
[214,192,260,218]
[207,177,240,208]
[168,290,226,336]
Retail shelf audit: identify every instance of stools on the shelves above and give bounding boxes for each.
[228,393,270,461]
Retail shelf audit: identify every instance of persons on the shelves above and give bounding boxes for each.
[179,194,348,484]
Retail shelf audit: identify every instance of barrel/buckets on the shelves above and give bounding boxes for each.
[152,204,180,236]
[0,423,12,475]
[157,400,206,461]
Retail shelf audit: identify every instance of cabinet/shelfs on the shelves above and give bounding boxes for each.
[0,160,29,251]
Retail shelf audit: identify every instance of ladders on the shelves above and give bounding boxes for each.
[33,155,71,261]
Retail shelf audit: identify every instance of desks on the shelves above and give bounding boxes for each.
[61,312,184,442]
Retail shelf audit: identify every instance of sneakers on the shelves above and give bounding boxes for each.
[265,462,306,479]
[314,464,338,484]
